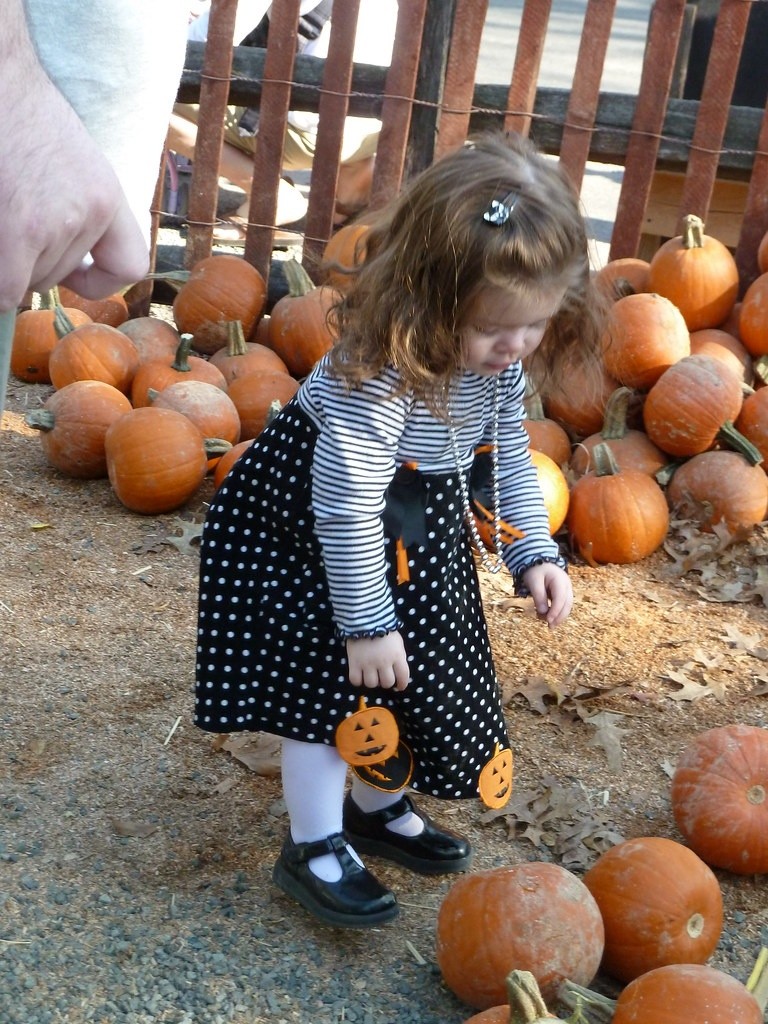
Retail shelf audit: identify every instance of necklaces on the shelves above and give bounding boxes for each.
[436,376,507,576]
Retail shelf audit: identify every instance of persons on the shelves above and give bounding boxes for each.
[193,133,614,935]
[1,0,191,439]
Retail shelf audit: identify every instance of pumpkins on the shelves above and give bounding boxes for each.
[9,221,378,513]
[470,213,768,568]
[436,724,768,1024]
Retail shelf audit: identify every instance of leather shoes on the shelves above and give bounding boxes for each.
[272,825,399,927]
[341,790,471,875]
